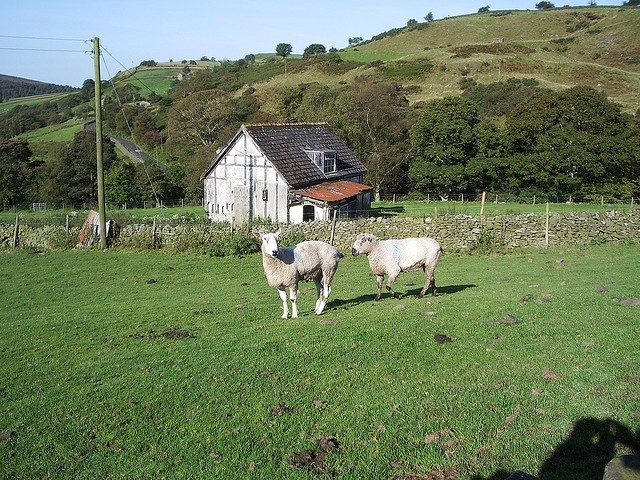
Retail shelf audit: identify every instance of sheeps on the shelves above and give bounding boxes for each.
[252,227,345,318]
[351,233,443,300]
